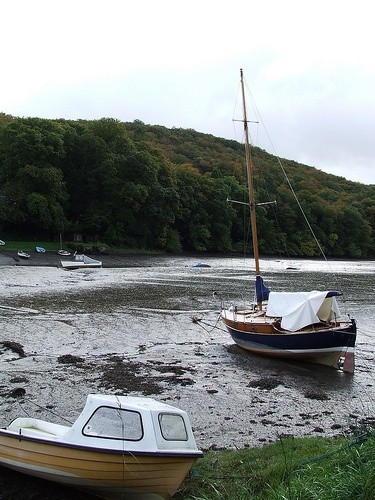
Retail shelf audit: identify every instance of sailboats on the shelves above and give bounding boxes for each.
[218,67,357,375]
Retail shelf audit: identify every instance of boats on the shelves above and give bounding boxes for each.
[60,254,103,269]
[0,392,204,500]
[36,246,46,253]
[57,250,71,256]
[17,250,30,258]
[0,240,6,246]
